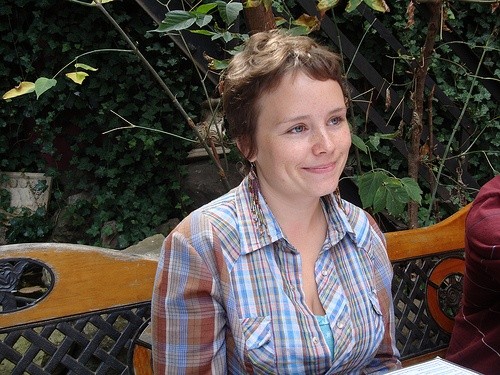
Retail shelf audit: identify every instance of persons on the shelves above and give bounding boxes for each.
[150,29,403,375]
[446,171,500,375]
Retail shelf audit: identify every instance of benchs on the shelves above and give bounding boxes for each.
[0,198,473,375]
